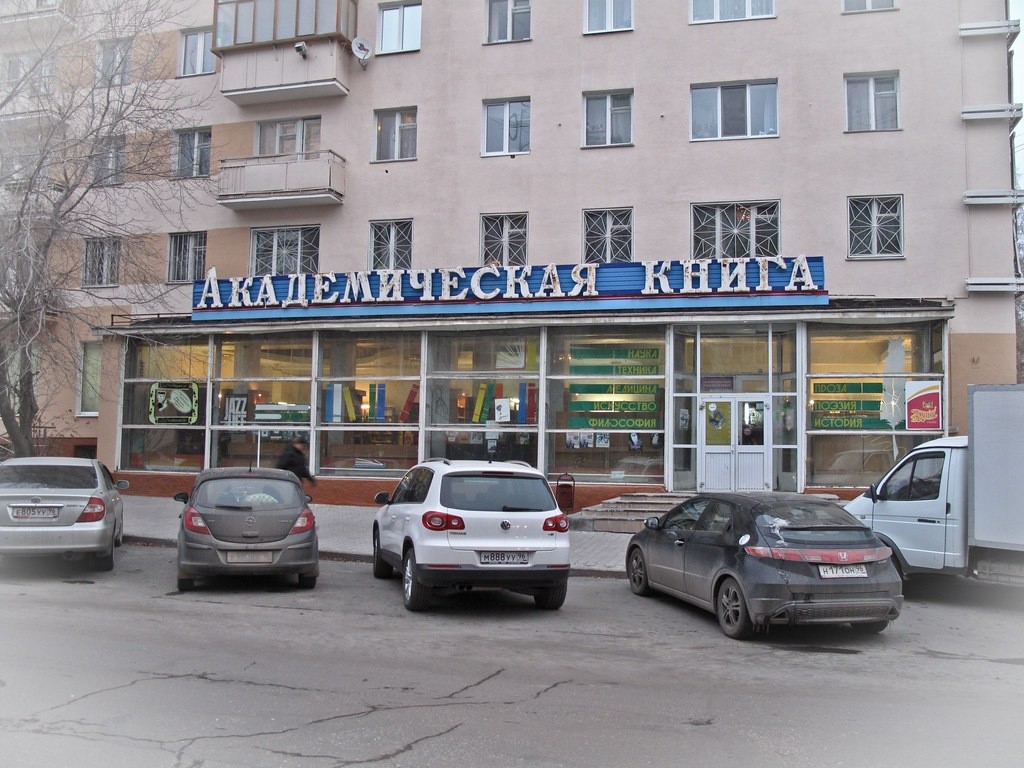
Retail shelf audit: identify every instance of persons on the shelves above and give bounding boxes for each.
[275,436,316,486]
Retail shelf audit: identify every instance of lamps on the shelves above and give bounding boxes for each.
[295,42,307,57]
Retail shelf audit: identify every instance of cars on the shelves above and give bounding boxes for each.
[625,491,903,641]
[0,457,129,572]
[173,468,320,590]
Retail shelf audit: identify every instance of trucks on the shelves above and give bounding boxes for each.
[810,449,893,487]
[842,383,1024,594]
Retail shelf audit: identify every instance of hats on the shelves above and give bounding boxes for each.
[293,435,307,444]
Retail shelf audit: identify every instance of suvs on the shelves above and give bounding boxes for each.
[607,458,664,484]
[372,457,571,611]
